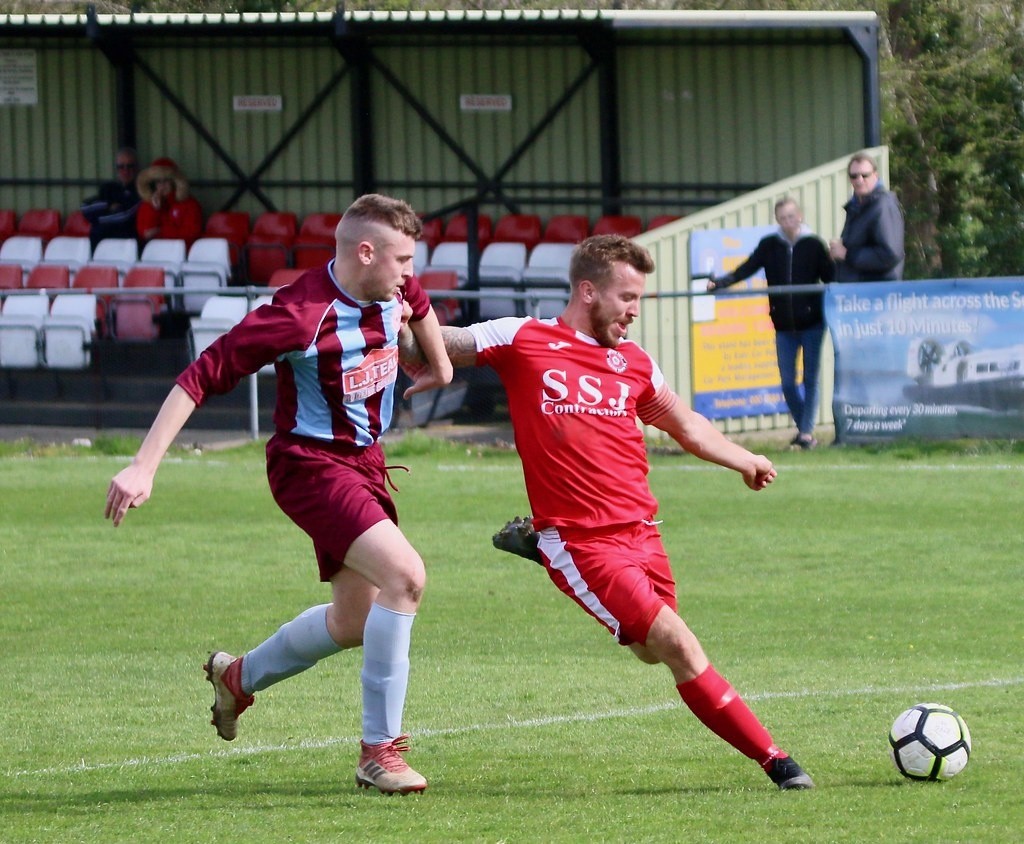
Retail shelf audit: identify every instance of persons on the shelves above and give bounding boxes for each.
[79,149,140,250]
[706,199,833,449]
[831,154,905,283]
[103,194,452,797]
[404,235,811,790]
[136,157,202,247]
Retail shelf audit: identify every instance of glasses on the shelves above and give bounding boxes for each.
[846,168,874,180]
[113,162,136,172]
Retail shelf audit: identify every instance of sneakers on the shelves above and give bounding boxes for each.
[202,652,256,742]
[765,755,815,790]
[354,736,429,797]
[491,516,548,567]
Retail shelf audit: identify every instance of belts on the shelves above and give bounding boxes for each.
[275,430,367,455]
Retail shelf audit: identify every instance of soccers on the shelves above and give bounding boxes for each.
[888,702,971,781]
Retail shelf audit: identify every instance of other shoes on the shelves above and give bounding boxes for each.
[786,434,819,451]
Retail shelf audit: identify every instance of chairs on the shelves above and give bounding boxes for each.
[0,202,684,373]
